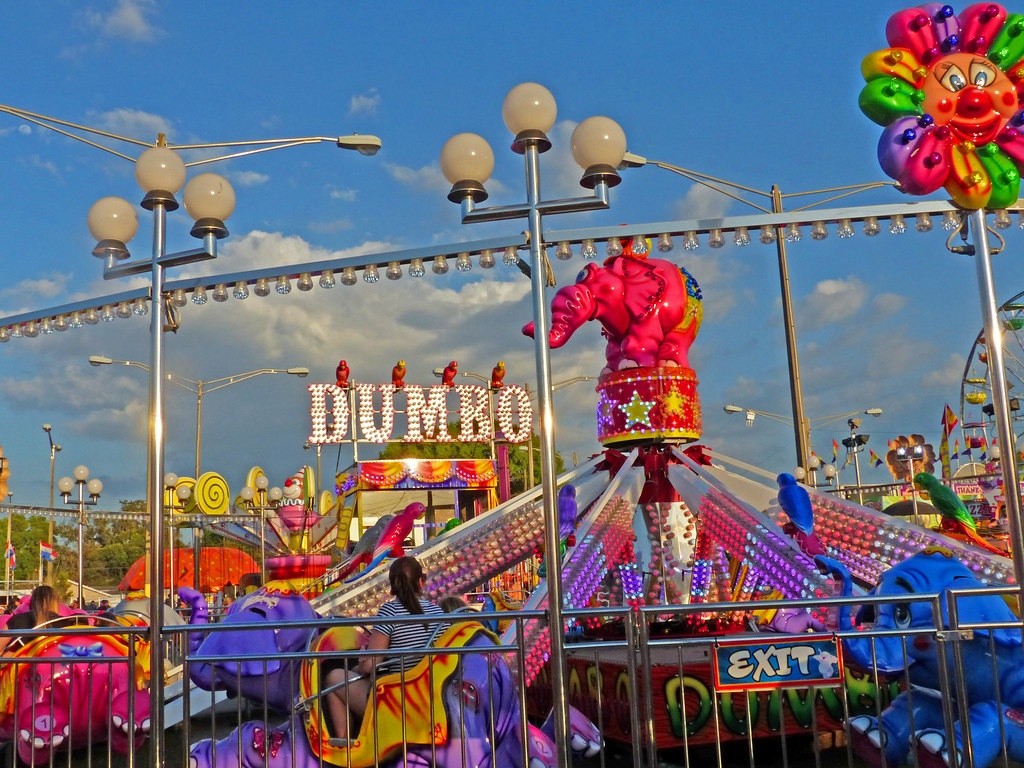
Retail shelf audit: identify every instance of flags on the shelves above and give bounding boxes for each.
[41,542,58,562]
[4,540,15,569]
[939,405,958,488]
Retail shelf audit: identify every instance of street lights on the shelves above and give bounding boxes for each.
[86,148,238,768]
[793,457,838,491]
[2,106,384,598]
[87,354,311,590]
[58,465,103,608]
[442,82,627,767]
[724,403,883,486]
[42,423,62,590]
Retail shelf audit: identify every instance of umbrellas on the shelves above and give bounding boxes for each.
[882,499,939,523]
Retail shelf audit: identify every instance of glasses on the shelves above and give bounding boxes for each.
[421,574,426,583]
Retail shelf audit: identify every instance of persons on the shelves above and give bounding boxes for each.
[70,613,89,625]
[437,597,469,614]
[165,594,191,620]
[4,598,20,615]
[219,581,241,622]
[324,556,451,739]
[71,596,111,611]
[94,612,119,626]
[0,585,77,668]
